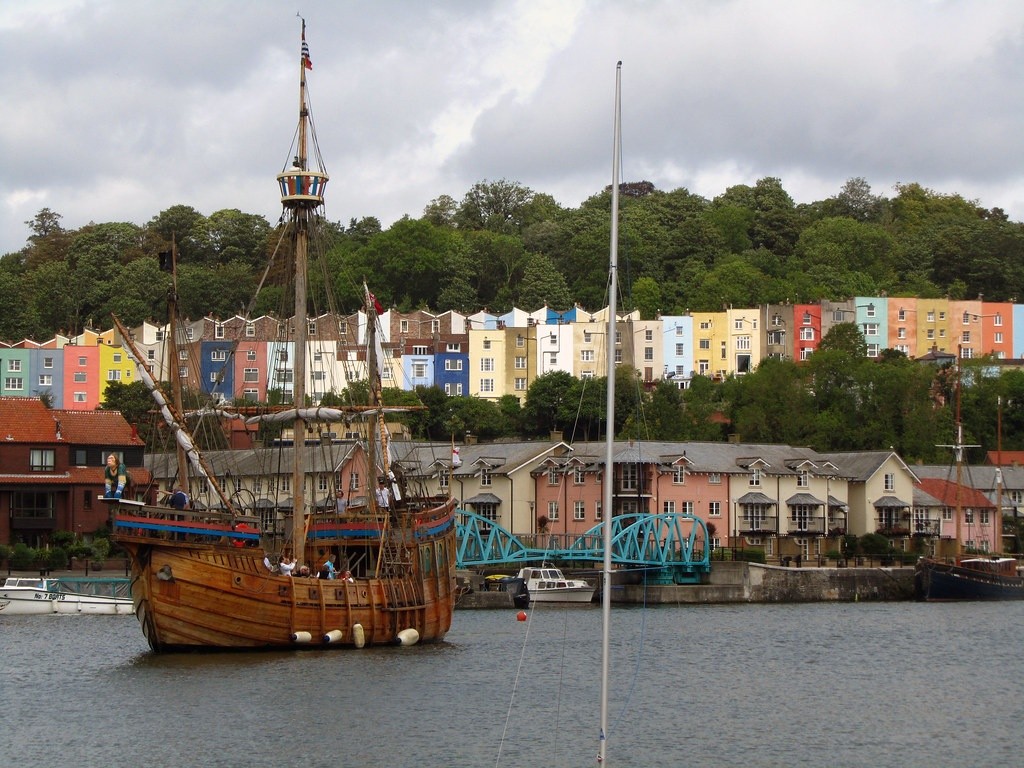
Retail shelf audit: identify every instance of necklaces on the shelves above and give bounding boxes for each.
[110,467,117,473]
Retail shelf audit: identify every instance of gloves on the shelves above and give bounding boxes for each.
[104,483,113,498]
[114,483,126,498]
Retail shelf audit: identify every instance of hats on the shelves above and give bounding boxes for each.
[379,480,385,484]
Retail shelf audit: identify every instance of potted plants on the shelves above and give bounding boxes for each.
[90,538,110,572]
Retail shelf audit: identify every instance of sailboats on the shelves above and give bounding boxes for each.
[915,342,1024,601]
[98,15,462,656]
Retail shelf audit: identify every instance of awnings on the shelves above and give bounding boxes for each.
[461,493,501,504]
[432,494,459,505]
[828,494,847,507]
[192,496,370,509]
[873,496,911,508]
[738,492,777,505]
[786,493,826,506]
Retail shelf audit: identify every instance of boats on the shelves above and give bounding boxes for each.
[475,574,531,608]
[516,564,597,603]
[0,576,136,616]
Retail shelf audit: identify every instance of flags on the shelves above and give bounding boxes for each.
[301,36,312,70]
[368,290,383,315]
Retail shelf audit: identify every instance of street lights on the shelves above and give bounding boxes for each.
[843,506,850,567]
[966,509,972,555]
[526,501,536,548]
[732,495,739,560]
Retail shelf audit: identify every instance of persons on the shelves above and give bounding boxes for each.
[324,555,336,579]
[343,571,353,583]
[259,548,272,570]
[278,557,298,576]
[407,572,412,576]
[316,565,331,579]
[295,566,315,578]
[334,490,351,541]
[106,453,133,499]
[398,573,403,579]
[482,578,497,591]
[155,485,189,540]
[371,482,394,539]
